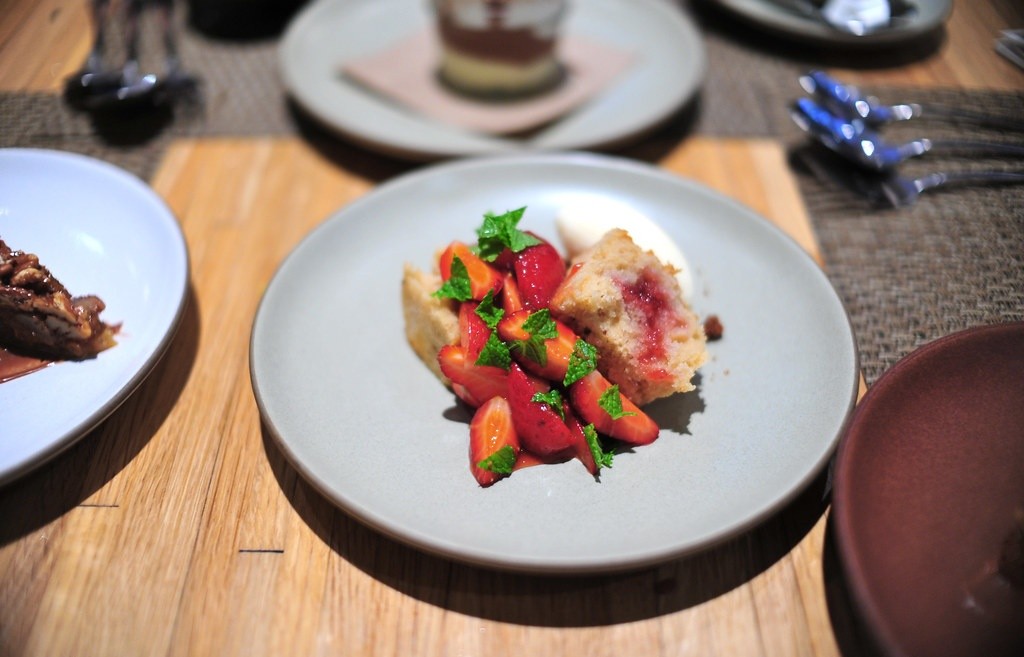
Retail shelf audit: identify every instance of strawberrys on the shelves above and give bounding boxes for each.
[434,229,660,491]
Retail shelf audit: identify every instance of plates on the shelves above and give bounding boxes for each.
[247,152,858,576]
[834,321,1024,657]
[0,147,189,486]
[718,0,954,43]
[275,0,707,153]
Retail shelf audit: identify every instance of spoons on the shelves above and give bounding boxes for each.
[65,0,202,112]
[790,70,1024,209]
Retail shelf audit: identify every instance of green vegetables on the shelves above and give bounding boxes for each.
[430,204,637,479]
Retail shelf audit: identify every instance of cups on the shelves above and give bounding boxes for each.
[433,1,566,101]
